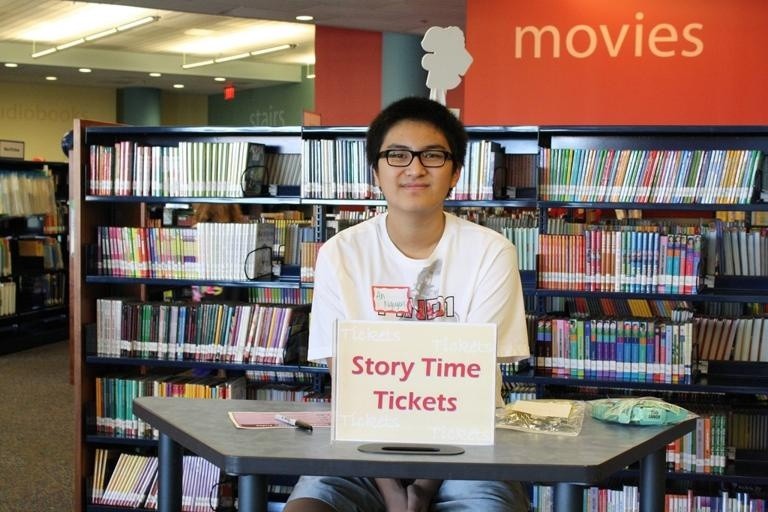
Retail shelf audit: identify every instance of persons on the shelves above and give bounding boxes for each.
[282,97,531,512]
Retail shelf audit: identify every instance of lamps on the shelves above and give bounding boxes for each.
[31,3,161,59]
[180,41,298,69]
[223,79,236,102]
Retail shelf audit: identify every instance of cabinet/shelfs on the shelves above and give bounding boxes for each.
[0,158,70,356]
[66,125,768,512]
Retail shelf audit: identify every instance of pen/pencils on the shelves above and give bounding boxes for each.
[274,415,313,431]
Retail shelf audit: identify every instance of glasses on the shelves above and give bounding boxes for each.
[375,147,457,169]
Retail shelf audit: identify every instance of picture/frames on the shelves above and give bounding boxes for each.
[0,139,25,161]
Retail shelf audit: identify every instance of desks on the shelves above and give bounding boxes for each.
[130,396,698,512]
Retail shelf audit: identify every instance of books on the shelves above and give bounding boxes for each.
[87,139,759,204]
[96,286,768,385]
[91,370,767,511]
[96,205,767,294]
